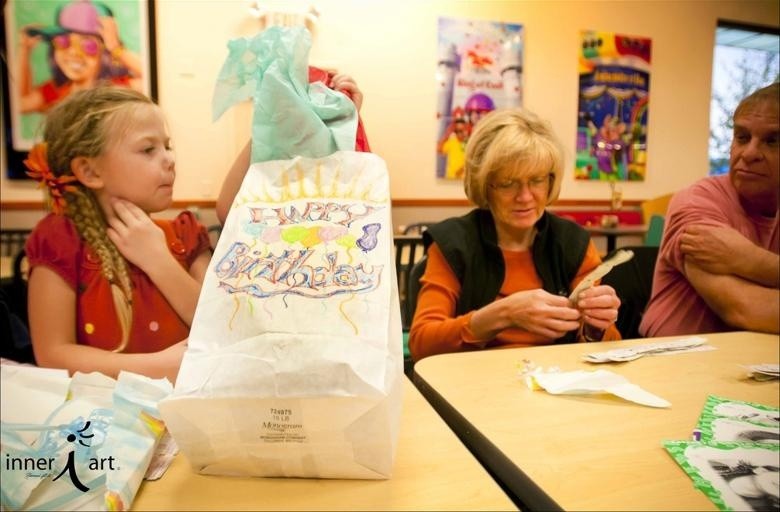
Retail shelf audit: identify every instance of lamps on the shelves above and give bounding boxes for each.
[10,248,37,369]
[1,228,36,256]
[396,222,433,294]
[408,253,430,329]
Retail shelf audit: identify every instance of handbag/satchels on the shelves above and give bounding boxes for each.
[111,45,124,67]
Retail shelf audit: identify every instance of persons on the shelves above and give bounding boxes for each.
[638,81,780,337]
[407,108,623,361]
[24,86,215,381]
[437,117,467,179]
[18,0,143,113]
[214,70,364,225]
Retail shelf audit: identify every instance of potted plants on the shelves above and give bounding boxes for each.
[0,1,160,180]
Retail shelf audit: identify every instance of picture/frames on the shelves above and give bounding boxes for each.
[122,372,526,512]
[583,223,649,264]
[392,234,425,246]
[411,328,780,511]
